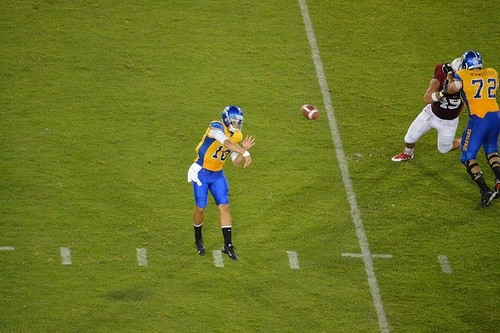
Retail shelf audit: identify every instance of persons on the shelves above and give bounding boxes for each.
[187,105,255,261]
[391,58,464,161]
[447,50,500,206]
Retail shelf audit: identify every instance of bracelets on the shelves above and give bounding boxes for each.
[432,92,439,102]
[243,151,251,157]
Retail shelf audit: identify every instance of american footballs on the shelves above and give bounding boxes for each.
[301,104,319,120]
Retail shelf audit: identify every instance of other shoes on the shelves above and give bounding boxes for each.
[480,188,497,206]
[493,178,500,199]
[391,151,414,162]
[196,242,205,256]
[221,246,237,262]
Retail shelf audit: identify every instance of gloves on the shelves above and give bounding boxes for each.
[442,63,452,76]
[440,79,449,98]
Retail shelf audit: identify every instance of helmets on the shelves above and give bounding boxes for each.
[451,57,463,72]
[463,49,482,69]
[221,106,244,132]
